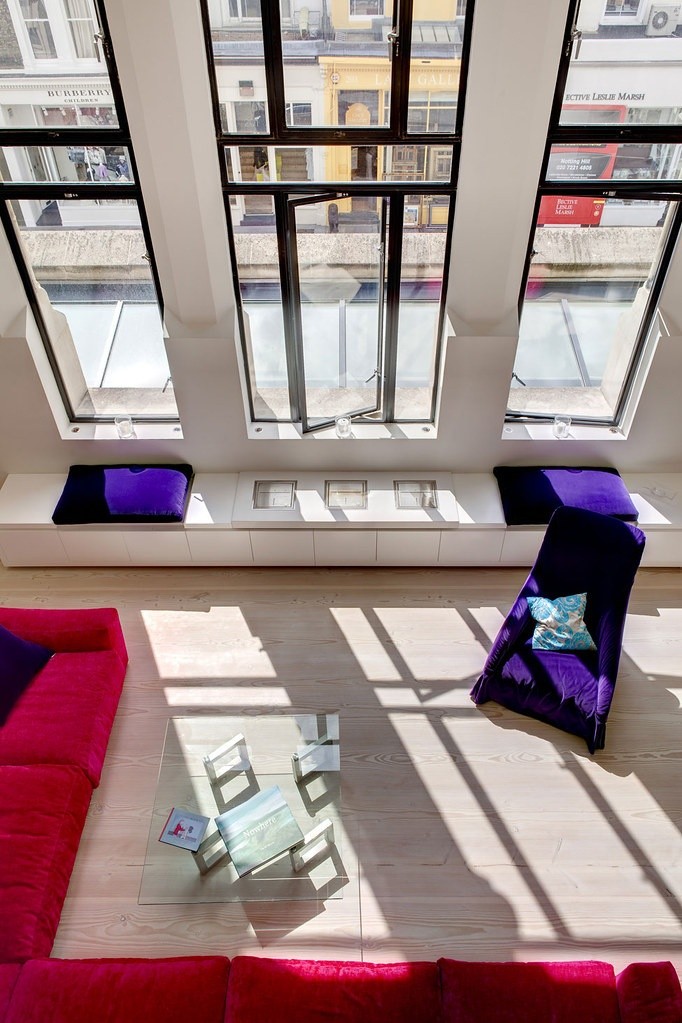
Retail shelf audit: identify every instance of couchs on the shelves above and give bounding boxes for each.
[0,956,682,1023]
[0,607,127,963]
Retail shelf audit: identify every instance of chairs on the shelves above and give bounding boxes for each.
[460,504,648,758]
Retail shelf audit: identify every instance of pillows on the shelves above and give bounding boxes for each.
[525,592,598,652]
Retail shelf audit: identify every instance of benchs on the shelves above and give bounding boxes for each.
[0,470,682,568]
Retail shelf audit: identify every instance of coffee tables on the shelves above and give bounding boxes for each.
[136,713,344,905]
